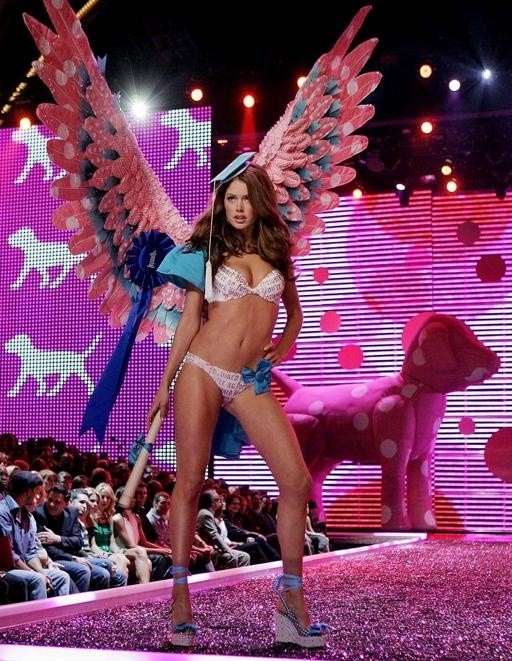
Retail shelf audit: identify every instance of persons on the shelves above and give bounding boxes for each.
[147,161,327,648]
[1,433,176,605]
[188,478,331,576]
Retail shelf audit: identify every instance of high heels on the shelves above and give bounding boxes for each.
[171,622,196,646]
[275,591,333,648]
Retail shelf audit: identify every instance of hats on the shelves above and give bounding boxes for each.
[14,471,42,486]
